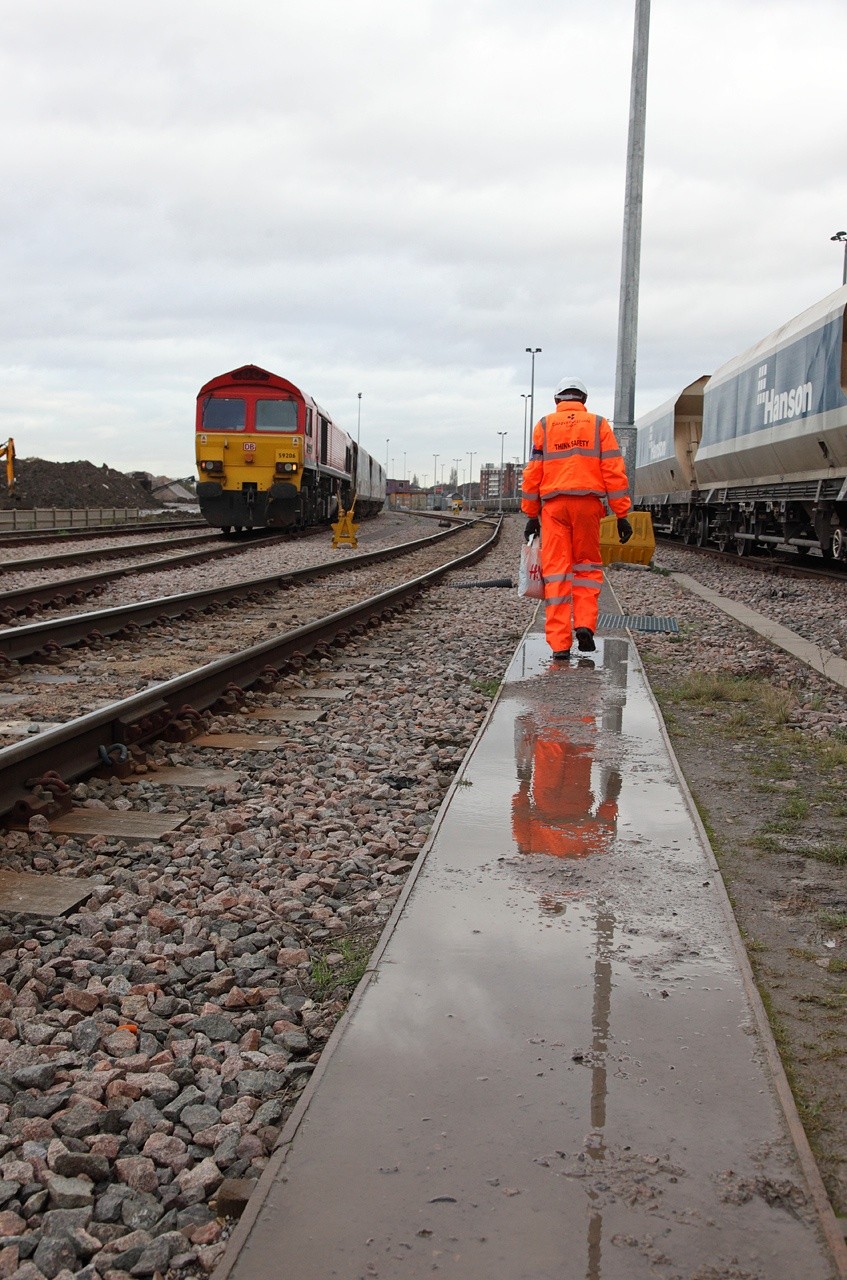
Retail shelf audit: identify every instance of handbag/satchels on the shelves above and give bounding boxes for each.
[518,533,545,600]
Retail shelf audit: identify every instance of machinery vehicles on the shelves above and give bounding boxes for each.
[0,437,34,510]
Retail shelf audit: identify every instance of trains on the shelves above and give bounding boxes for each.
[634,284,847,574]
[196,364,387,537]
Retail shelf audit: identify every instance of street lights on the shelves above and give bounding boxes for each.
[466,452,477,512]
[357,391,363,445]
[520,393,531,469]
[452,458,462,493]
[830,230,847,287]
[440,464,445,495]
[404,451,407,480]
[386,438,390,475]
[526,348,542,460]
[497,432,507,513]
[433,454,440,508]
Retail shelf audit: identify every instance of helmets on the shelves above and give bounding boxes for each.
[556,377,588,396]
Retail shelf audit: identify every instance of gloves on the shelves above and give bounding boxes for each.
[524,518,541,540]
[617,518,633,544]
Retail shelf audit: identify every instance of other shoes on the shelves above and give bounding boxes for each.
[552,650,570,658]
[574,628,596,652]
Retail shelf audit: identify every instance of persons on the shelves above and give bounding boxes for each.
[520,375,634,660]
[511,659,623,918]
